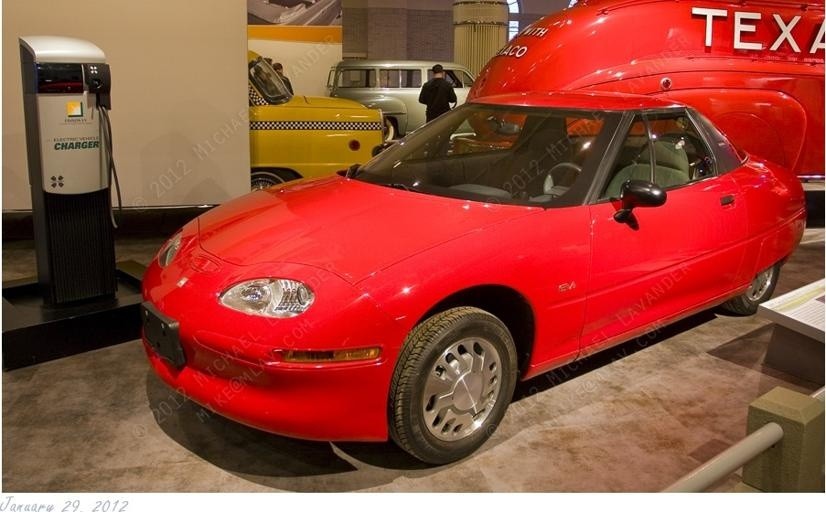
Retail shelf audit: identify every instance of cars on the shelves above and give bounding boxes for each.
[139,88,809,467]
[247,49,385,193]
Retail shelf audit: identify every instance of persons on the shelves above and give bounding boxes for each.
[419,63,457,157]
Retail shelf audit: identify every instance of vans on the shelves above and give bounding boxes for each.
[327,59,475,139]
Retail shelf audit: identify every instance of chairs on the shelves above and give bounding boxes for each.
[503,129,579,193]
[604,142,690,198]
